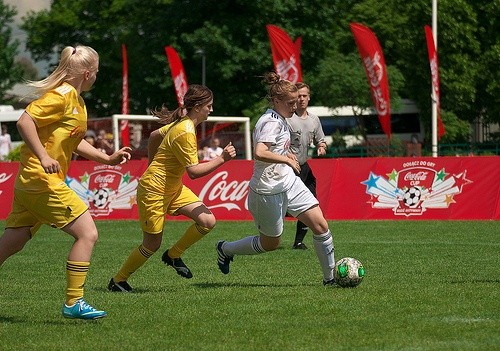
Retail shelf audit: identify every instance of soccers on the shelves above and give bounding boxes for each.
[333,257,366,288]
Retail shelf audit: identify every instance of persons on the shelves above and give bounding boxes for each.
[0,46,132,320]
[285,82,327,251]
[215,71,337,286]
[108,85,236,292]
[0,125,224,161]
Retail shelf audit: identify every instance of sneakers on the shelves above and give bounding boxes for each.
[323,278,339,286]
[292,242,308,250]
[215,241,234,274]
[108,278,138,294]
[162,249,193,278]
[62,297,107,320]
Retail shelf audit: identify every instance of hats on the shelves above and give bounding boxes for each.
[83,130,97,142]
